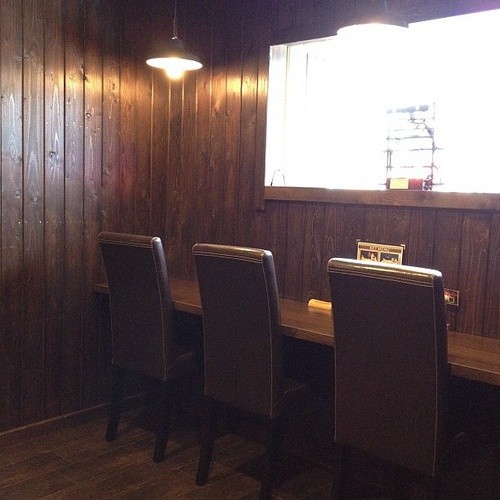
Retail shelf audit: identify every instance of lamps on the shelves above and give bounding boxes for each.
[146,1,204,82]
[337,1,410,43]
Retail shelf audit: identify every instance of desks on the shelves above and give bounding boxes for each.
[92,275,500,388]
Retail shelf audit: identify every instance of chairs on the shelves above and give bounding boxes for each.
[327,257,481,500]
[98,232,202,464]
[192,243,332,500]
